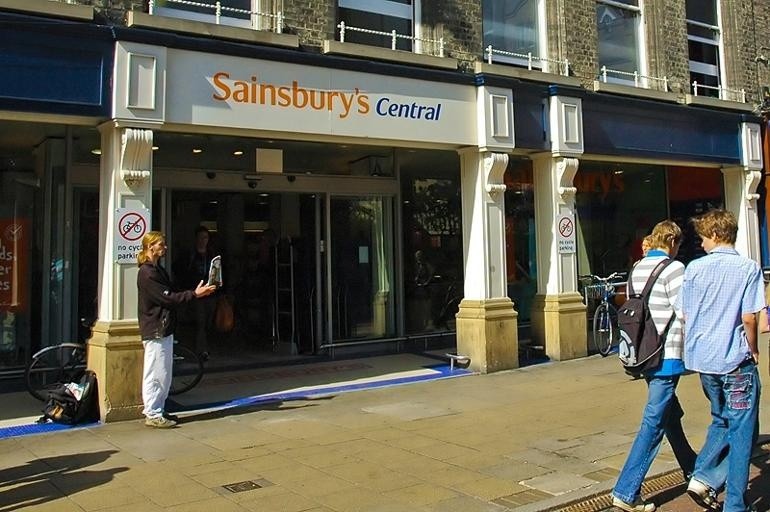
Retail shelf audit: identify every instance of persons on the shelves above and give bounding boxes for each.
[641,234,652,256]
[612,219,719,512]
[680,208,766,512]
[182,224,223,362]
[136,230,216,428]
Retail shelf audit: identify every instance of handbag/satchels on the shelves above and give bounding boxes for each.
[214,293,234,333]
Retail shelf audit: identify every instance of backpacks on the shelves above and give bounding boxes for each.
[43,370,98,425]
[617,256,675,379]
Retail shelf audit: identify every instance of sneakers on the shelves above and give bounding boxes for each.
[686,477,722,512]
[145,414,179,428]
[611,491,656,512]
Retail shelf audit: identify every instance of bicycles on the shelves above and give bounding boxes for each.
[25,318,210,401]
[578,271,625,358]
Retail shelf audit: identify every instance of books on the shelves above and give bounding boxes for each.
[206,255,222,288]
